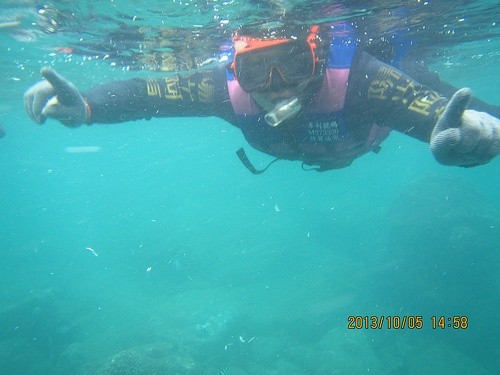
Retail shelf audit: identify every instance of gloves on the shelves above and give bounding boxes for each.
[23,67,86,128]
[430,87,500,167]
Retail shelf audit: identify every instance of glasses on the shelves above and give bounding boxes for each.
[230,37,316,90]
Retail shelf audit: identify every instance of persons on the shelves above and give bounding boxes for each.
[23,19,500,166]
